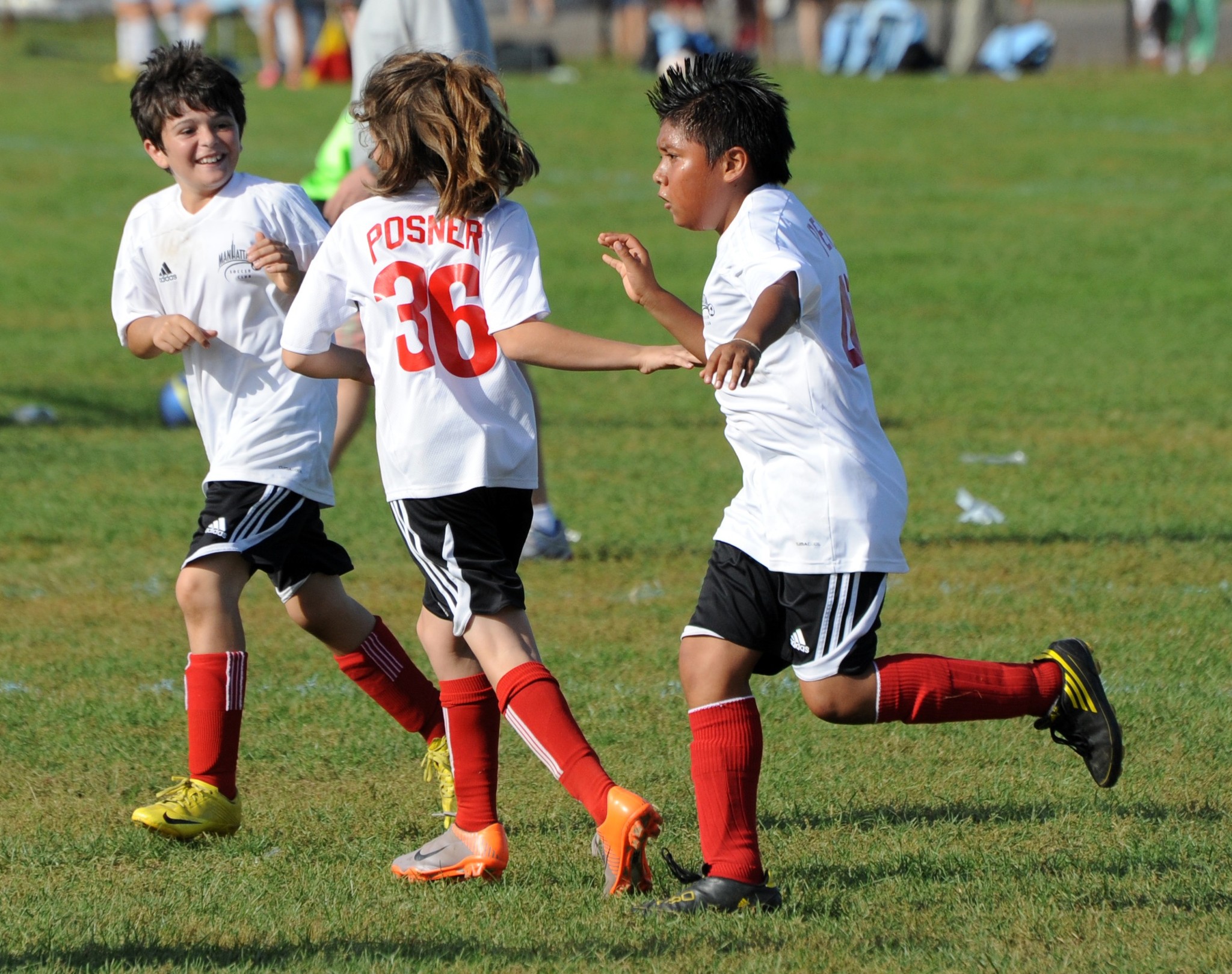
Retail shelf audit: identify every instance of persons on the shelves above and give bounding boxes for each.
[598,51,1124,916]
[109,37,458,839]
[277,50,710,901]
[295,0,571,562]
[102,0,1223,80]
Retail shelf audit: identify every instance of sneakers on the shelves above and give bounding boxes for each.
[519,520,573,560]
[631,846,783,917]
[421,735,458,829]
[1033,637,1125,788]
[391,823,509,883]
[131,775,243,842]
[591,786,663,898]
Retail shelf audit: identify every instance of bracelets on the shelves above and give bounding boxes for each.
[730,338,762,360]
